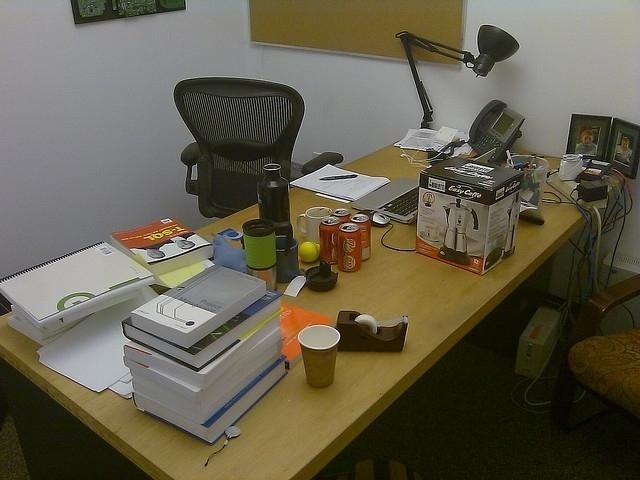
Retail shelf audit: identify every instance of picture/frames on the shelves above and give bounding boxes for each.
[605,118,640,178]
[566,114,612,162]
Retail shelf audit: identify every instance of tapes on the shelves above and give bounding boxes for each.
[354,313,377,337]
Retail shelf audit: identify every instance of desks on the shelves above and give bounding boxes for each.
[0,142,624,480]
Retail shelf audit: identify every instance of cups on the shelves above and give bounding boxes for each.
[296,206,332,243]
[297,324,341,389]
[241,218,278,291]
[275,235,298,283]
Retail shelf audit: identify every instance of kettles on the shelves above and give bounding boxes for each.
[213,228,248,275]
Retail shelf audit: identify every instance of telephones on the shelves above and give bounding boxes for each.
[466,99,525,160]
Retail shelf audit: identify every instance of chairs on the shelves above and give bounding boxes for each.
[549,271,640,431]
[171,75,344,219]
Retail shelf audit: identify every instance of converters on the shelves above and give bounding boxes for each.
[577,179,608,203]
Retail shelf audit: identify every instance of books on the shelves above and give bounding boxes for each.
[121,265,290,446]
[109,217,215,277]
[1,240,155,346]
[289,163,391,202]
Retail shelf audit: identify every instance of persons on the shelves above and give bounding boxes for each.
[575,130,597,157]
[615,136,632,164]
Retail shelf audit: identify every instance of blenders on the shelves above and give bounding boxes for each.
[437,197,479,266]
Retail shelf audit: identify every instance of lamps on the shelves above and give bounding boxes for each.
[394,23,520,131]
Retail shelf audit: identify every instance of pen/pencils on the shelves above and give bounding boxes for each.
[320,174,358,180]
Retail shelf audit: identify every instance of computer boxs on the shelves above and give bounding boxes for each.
[514,305,562,379]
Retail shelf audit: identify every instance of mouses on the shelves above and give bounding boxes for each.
[358,209,391,227]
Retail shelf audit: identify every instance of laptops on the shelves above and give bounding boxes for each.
[351,148,497,226]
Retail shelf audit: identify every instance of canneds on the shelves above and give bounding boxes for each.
[319,217,340,265]
[349,213,371,262]
[338,223,361,272]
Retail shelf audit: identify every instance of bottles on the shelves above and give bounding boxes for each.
[246,263,277,292]
[257,164,290,235]
[242,219,277,270]
[333,209,351,223]
